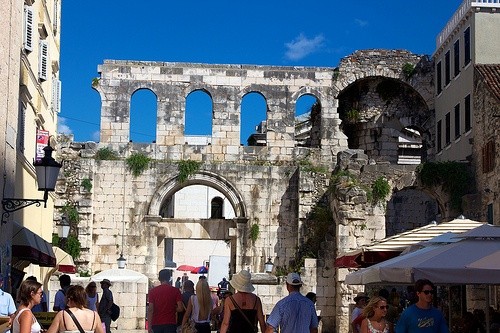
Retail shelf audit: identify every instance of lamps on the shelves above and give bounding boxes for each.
[116,253,127,269]
[264,259,274,273]
[0,146,63,224]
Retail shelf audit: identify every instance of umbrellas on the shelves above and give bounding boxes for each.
[344,224,500,333]
[176,265,209,275]
[334,215,500,268]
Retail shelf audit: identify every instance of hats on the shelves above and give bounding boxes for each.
[100,279,111,286]
[229,269,255,293]
[354,292,370,302]
[286,273,302,285]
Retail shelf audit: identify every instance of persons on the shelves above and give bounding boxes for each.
[220,270,266,333]
[357,296,394,333]
[266,273,318,333]
[179,279,217,333]
[148,269,182,333]
[395,279,448,333]
[0,276,16,333]
[46,286,103,333]
[26,276,113,333]
[12,280,43,333]
[305,292,322,324]
[350,288,500,333]
[175,273,229,333]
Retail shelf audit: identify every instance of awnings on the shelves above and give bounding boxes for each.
[12,222,76,273]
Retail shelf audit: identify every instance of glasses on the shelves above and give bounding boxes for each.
[422,290,434,295]
[36,293,44,298]
[91,286,96,288]
[380,305,388,310]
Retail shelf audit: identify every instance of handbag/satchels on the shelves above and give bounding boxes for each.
[194,322,211,333]
[110,303,120,321]
